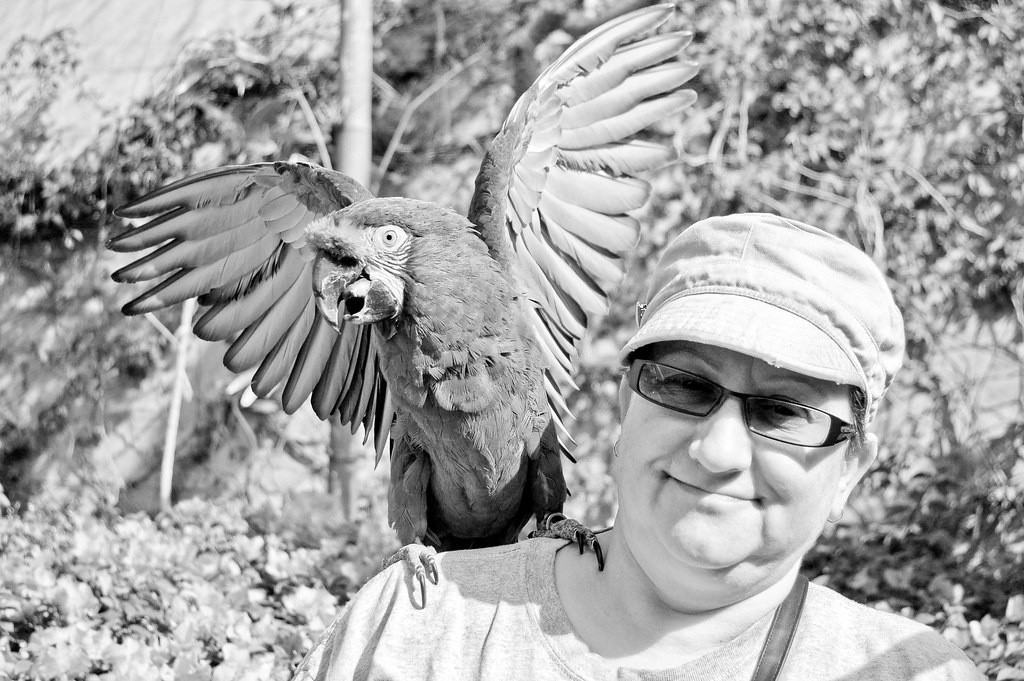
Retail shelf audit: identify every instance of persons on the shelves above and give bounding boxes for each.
[289,213,989,681]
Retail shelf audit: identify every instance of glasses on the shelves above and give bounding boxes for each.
[627,359,856,448]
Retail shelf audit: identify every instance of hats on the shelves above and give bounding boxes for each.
[619,213,905,428]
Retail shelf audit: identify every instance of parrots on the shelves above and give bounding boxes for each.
[101,1,701,611]
